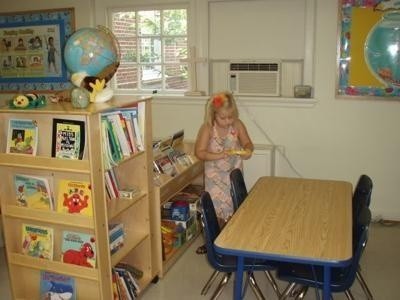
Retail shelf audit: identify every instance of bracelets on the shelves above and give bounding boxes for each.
[244,143,254,152]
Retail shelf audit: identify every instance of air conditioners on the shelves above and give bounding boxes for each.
[225,59,282,96]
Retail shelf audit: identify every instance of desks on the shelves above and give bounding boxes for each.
[211,175,356,300]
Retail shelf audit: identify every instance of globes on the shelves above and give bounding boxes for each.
[63,25,121,93]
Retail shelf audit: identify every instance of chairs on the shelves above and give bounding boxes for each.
[229,166,249,214]
[351,173,373,217]
[275,208,375,300]
[198,189,281,300]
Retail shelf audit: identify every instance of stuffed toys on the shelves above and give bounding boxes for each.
[5,70,113,108]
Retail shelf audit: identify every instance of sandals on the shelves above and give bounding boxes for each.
[197,244,207,254]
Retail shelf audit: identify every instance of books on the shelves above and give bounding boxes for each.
[4,111,201,299]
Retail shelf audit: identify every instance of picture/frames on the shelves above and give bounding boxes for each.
[0,6,78,95]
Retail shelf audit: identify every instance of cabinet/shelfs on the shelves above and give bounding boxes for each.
[0,88,160,298]
[153,137,207,275]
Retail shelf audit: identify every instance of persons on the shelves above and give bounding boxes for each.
[194,92,255,254]
[46,36,57,71]
